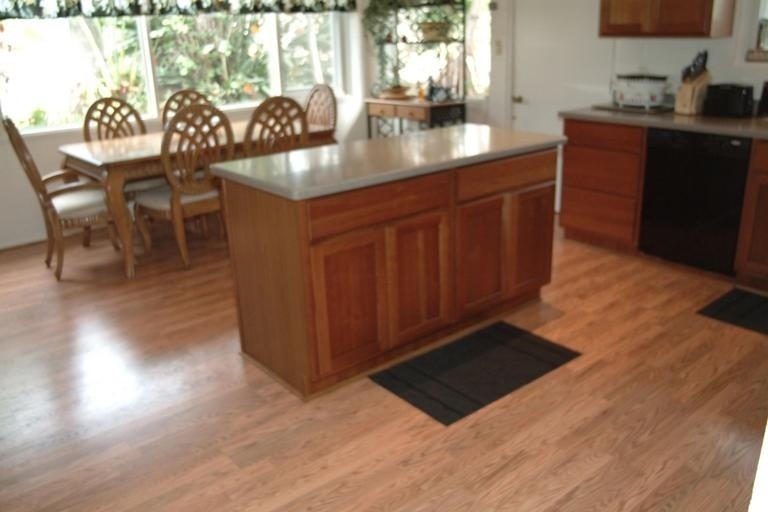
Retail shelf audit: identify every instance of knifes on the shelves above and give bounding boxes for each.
[679,48,710,82]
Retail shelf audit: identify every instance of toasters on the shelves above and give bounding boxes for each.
[704,84,756,117]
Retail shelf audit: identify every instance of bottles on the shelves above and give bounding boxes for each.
[417,86,426,104]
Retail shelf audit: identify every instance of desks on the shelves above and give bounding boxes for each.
[365,99,466,139]
[558,105,768,286]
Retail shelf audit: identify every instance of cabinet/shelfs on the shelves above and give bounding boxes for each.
[378,0,465,45]
[453,148,558,333]
[599,0,736,39]
[223,172,453,402]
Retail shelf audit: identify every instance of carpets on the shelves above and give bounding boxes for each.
[367,320,583,426]
[696,288,768,336]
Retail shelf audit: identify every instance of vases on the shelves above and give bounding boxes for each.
[418,22,451,40]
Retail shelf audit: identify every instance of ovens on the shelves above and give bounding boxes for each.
[639,121,755,278]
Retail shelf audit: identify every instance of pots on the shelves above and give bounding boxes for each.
[609,72,672,111]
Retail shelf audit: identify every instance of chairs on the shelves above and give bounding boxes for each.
[304,84,336,128]
[163,90,214,180]
[242,95,309,157]
[3,116,139,281]
[84,98,166,200]
[134,105,234,267]
